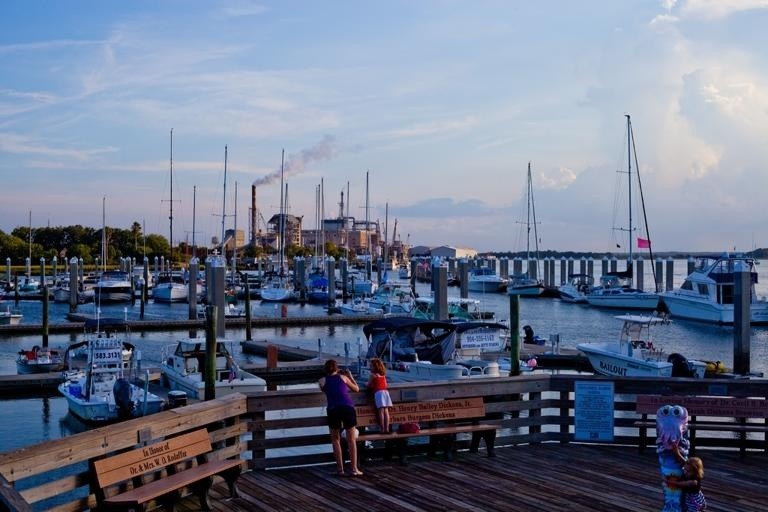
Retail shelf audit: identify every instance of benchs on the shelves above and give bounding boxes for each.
[634,394,766,456]
[355,398,497,457]
[93,427,245,512]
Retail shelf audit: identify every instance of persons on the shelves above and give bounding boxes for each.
[668,353,697,395]
[364,358,393,433]
[318,360,364,477]
[663,442,706,512]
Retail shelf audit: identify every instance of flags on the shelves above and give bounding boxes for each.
[637,238,650,248]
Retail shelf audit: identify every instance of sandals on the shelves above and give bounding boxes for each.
[351,470,363,476]
[330,470,345,476]
